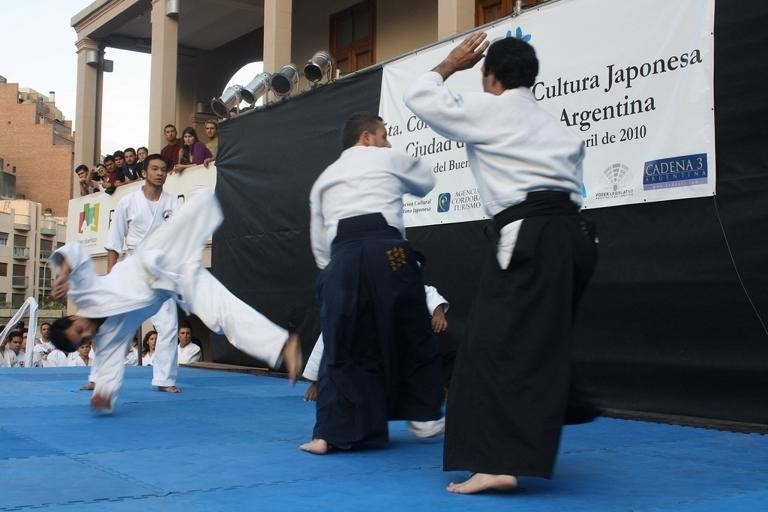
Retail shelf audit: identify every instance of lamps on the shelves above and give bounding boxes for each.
[210,50,337,120]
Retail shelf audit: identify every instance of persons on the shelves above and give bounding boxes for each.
[75,153,185,394]
[69,118,222,186]
[0,318,204,370]
[400,31,591,495]
[300,278,452,402]
[44,188,306,417]
[295,111,456,457]
